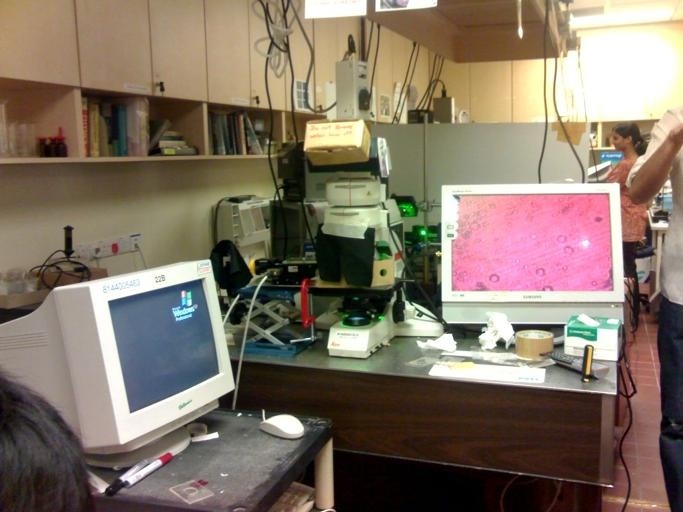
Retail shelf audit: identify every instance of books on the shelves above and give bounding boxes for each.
[208,112,264,156]
[149,118,199,155]
[81,96,128,157]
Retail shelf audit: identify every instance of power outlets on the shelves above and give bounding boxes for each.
[79,233,142,260]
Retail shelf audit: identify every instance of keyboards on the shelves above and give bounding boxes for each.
[429,364,546,387]
[268,482,315,512]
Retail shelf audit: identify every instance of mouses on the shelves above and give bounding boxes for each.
[259,414,304,440]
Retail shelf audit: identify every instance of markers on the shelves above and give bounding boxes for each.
[118,453,173,488]
[104,458,148,496]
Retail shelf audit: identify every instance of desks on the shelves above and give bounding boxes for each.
[217,314,618,510]
[83,405,338,509]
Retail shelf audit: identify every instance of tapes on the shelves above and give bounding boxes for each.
[515,329,553,360]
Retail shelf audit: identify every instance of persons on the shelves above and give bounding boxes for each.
[0,371,94,512]
[626,111,683,512]
[601,123,649,330]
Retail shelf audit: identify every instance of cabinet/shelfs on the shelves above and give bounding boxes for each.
[0,267,108,307]
[0,0,436,166]
[439,21,683,124]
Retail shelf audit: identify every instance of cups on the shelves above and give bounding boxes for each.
[9,121,36,156]
[0,99,9,157]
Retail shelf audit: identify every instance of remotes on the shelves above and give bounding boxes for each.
[538,351,609,380]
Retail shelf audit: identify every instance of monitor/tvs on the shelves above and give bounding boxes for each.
[441,183,624,344]
[0,259,235,470]
